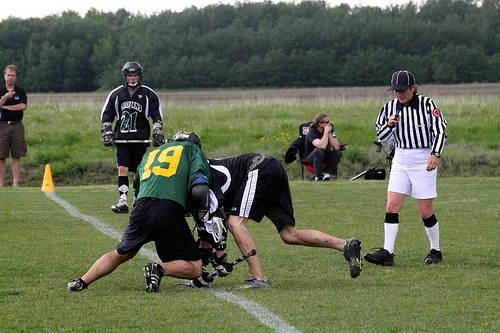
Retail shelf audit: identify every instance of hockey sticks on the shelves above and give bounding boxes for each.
[187,249,257,288]
[100,138,174,144]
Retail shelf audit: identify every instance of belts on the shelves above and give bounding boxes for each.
[0,120,21,126]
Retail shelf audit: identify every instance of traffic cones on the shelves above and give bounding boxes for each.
[42,164,56,191]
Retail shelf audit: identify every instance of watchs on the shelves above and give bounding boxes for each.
[431,152,440,158]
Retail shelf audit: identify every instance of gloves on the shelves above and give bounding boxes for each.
[208,251,233,278]
[151,124,165,147]
[196,238,213,266]
[101,123,115,148]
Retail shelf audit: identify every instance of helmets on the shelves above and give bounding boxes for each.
[173,130,201,149]
[122,61,143,89]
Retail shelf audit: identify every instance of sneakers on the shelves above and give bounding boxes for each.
[67,278,84,292]
[364,247,395,267]
[230,276,275,292]
[424,248,442,265]
[142,262,164,293]
[343,238,363,278]
[111,199,128,213]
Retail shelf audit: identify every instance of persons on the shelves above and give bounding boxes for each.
[187,152,363,290]
[100,61,165,213]
[68,130,211,292]
[0,65,28,188]
[364,70,448,267]
[304,113,340,181]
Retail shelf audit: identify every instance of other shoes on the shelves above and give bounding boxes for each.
[317,176,331,181]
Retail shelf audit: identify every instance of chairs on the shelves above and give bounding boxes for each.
[285,122,348,182]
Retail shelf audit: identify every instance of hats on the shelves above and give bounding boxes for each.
[388,70,414,91]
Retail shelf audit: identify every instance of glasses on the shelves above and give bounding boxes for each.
[320,121,329,124]
